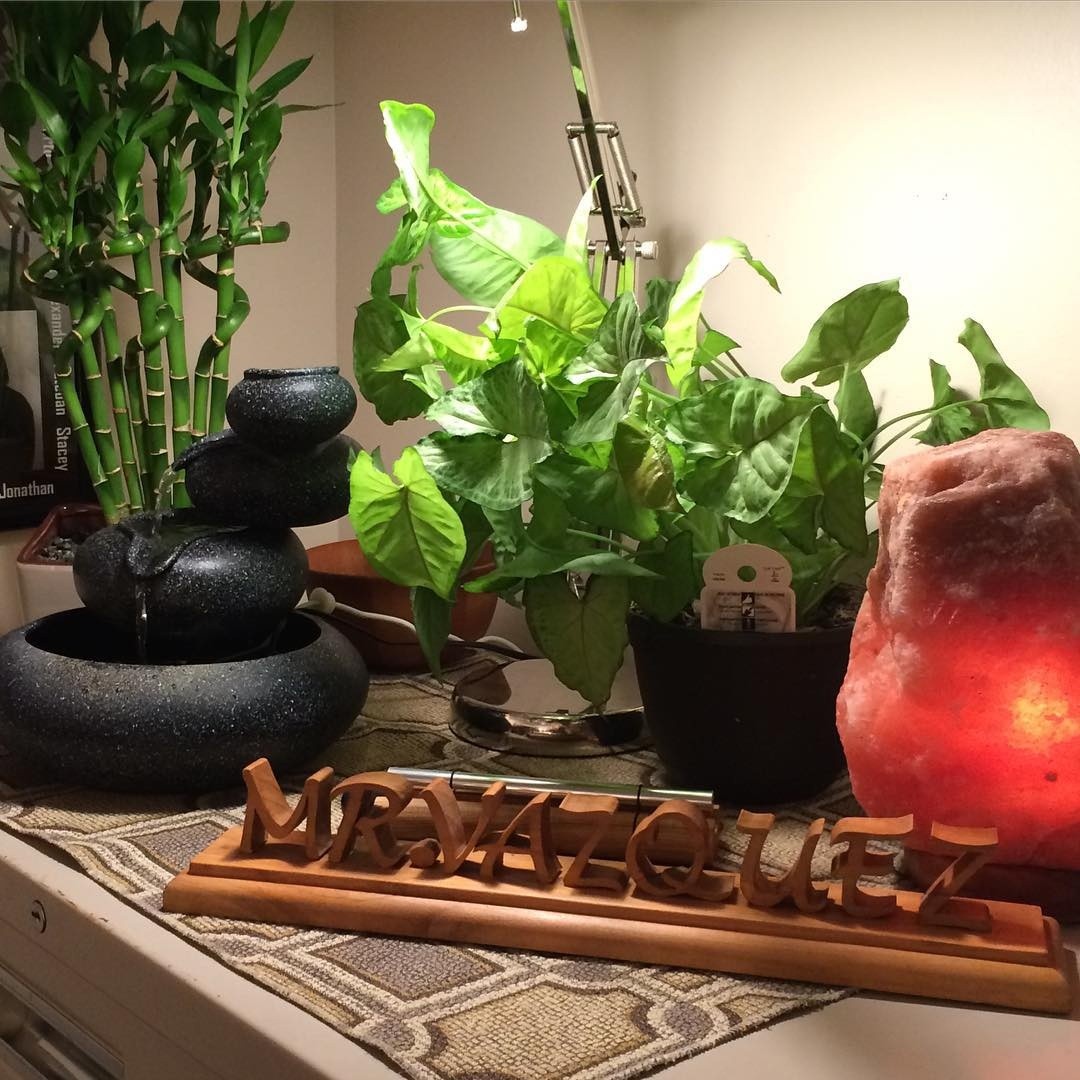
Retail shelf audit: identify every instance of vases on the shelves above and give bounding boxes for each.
[307,540,498,672]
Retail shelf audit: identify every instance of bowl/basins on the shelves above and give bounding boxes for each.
[303,539,500,666]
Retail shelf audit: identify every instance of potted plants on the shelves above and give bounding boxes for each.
[0,0,316,626]
[345,103,1052,810]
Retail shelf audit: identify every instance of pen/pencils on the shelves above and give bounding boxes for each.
[388,764,713,813]
